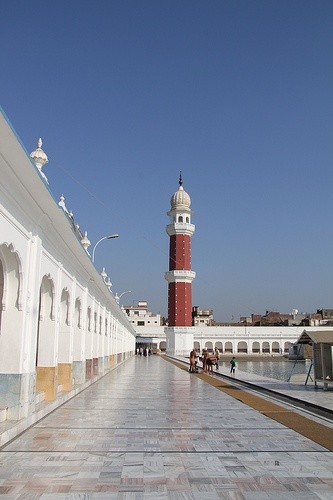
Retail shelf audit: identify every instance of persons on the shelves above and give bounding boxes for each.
[190,347,220,373]
[136,347,154,359]
[230,356,236,373]
